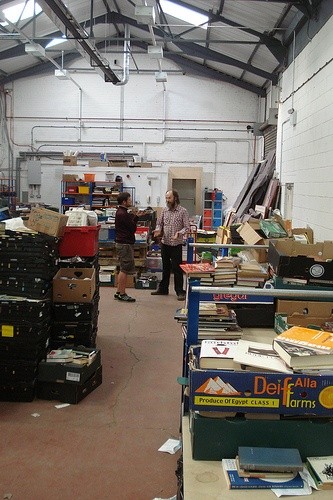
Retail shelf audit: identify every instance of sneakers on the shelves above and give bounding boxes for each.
[114,293,136,302]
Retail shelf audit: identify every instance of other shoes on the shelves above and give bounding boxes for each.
[151,290,169,295]
[177,293,186,301]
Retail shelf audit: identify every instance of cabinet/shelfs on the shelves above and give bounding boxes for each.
[202,190,222,231]
[60,180,122,216]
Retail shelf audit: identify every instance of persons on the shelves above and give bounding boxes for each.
[113,192,139,302]
[152,189,190,301]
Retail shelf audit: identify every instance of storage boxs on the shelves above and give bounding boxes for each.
[61,197,75,205]
[68,185,76,193]
[190,212,333,461]
[0,206,162,402]
[63,174,78,182]
[78,186,90,193]
[84,174,95,182]
[62,156,78,166]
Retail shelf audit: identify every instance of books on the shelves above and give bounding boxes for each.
[45,347,98,367]
[222,446,333,490]
[173,251,333,375]
[90,183,119,229]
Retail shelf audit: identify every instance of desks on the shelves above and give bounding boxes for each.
[180,244,333,500]
